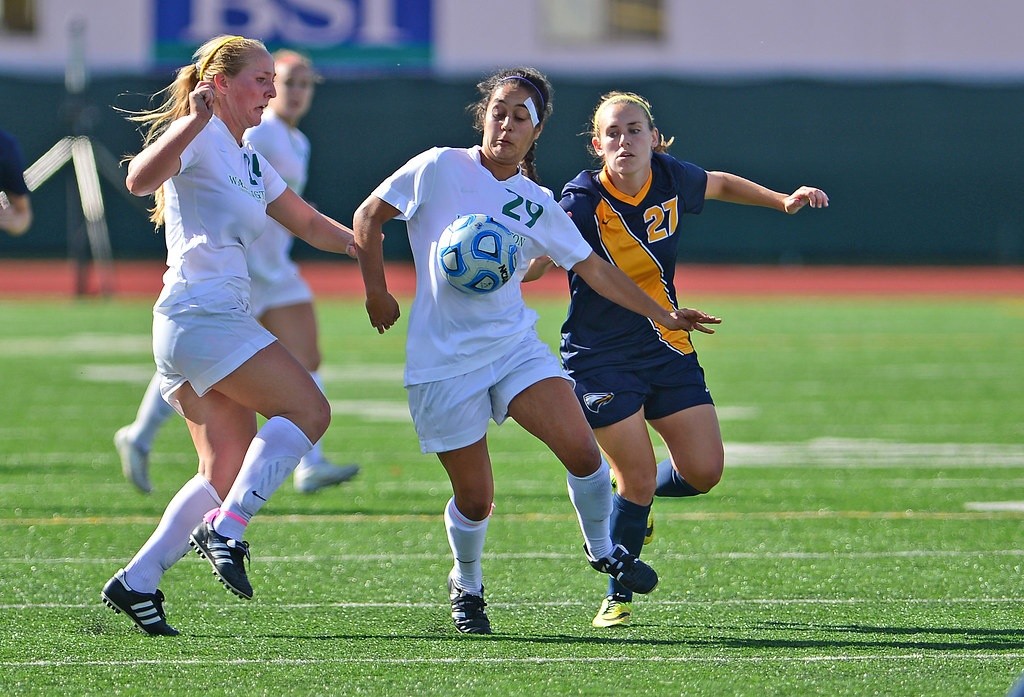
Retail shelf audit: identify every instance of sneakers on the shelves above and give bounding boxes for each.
[294,461,359,494]
[113,426,150,494]
[580,539,657,595]
[608,467,655,546]
[446,572,492,636]
[188,514,253,600]
[592,598,632,629]
[103,569,179,637]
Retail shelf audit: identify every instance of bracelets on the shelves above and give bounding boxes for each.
[346,237,358,256]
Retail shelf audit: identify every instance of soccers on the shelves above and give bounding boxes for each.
[436,213,518,296]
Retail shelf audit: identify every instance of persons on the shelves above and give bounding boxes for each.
[353,69,721,635]
[523,90,830,630]
[99,34,385,638]
